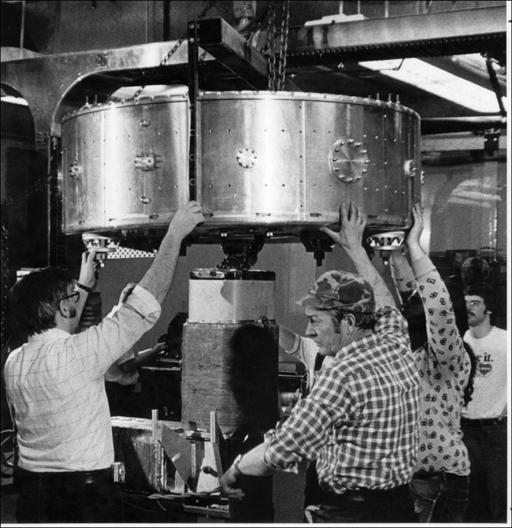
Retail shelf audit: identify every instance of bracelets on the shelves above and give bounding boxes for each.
[76,282,92,293]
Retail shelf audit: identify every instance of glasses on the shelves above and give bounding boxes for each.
[56,291,80,305]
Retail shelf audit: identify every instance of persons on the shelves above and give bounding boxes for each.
[461,250,488,271]
[400,202,477,523]
[219,199,421,523]
[277,322,329,524]
[461,285,508,523]
[4,200,206,523]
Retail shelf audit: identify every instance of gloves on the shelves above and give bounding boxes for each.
[219,453,248,502]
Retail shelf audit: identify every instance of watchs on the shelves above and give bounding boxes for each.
[233,454,243,476]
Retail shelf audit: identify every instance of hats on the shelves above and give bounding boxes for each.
[296,269,375,314]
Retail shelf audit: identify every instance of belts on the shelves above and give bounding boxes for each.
[326,484,409,505]
[14,465,113,484]
